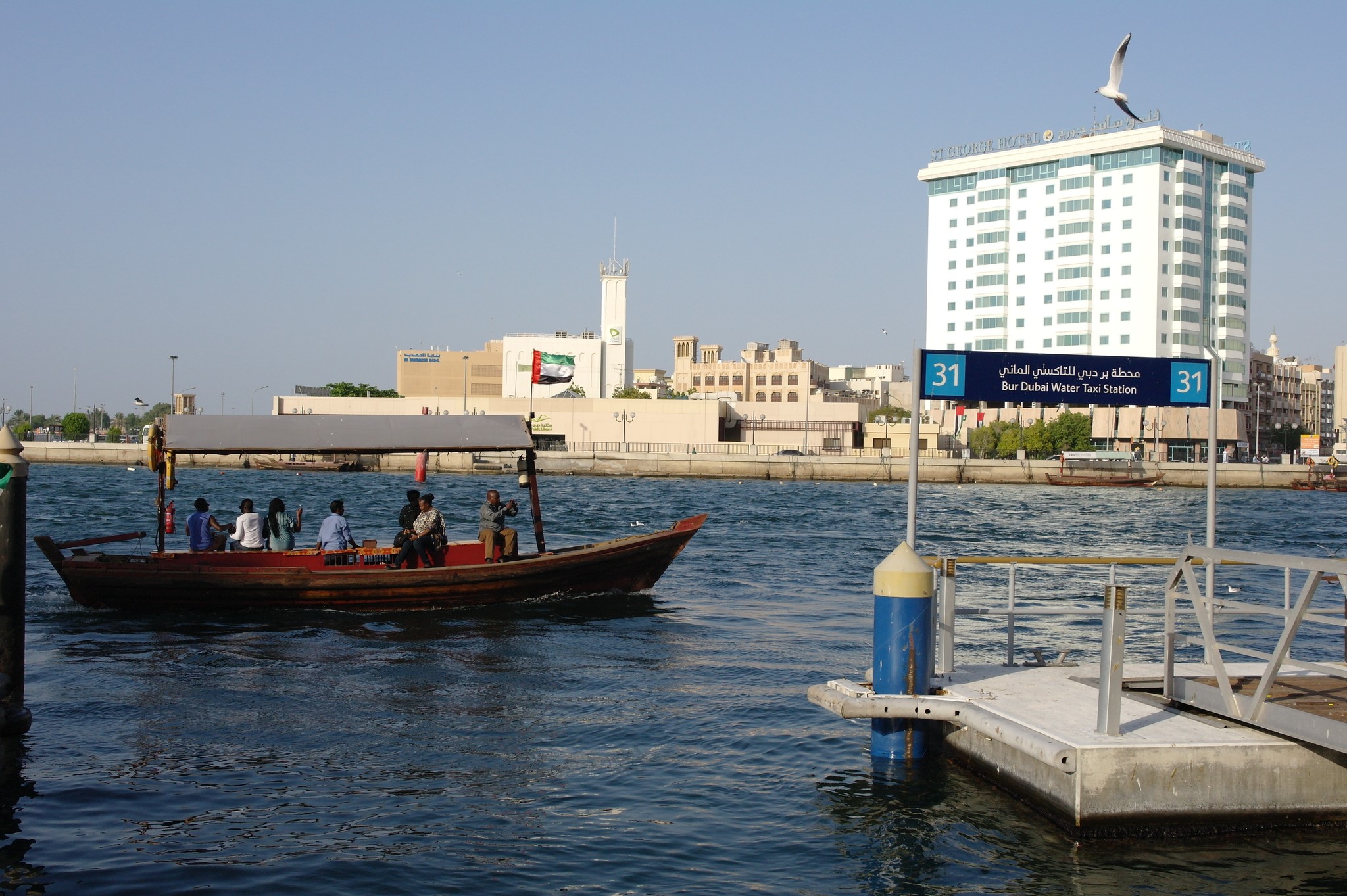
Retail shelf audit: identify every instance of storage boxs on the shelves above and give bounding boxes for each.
[363,539,377,548]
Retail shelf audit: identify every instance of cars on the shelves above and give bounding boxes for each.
[1201,453,1247,464]
[1043,454,1062,460]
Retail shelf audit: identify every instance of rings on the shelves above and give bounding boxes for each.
[411,540,412,541]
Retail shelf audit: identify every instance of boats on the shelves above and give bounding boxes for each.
[1291,455,1347,492]
[1044,450,1166,488]
[252,452,367,472]
[31,410,710,621]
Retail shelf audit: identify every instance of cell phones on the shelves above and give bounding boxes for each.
[299,504,301,510]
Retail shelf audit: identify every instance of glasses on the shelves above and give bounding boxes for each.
[204,503,210,507]
[239,506,246,509]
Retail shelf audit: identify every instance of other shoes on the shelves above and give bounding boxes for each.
[497,557,507,563]
[424,562,433,568]
[487,558,494,564]
[385,565,401,570]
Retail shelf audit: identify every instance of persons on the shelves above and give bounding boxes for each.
[1253,454,1269,464]
[384,493,445,570]
[478,490,519,564]
[316,500,361,565]
[262,498,303,551]
[228,499,264,550]
[1115,448,1121,462]
[1223,448,1229,463]
[692,447,697,454]
[186,498,233,552]
[399,490,433,568]
[24,429,31,441]
[1059,451,1065,462]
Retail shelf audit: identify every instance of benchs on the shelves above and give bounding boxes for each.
[150,540,503,570]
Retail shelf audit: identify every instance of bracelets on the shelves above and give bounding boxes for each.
[417,535,419,538]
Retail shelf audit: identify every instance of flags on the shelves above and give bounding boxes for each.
[531,350,576,384]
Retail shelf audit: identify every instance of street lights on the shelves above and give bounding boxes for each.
[250,385,269,415]
[875,412,898,457]
[175,386,196,414]
[888,394,903,407]
[1143,417,1167,462]
[1009,415,1033,460]
[86,403,104,443]
[742,411,765,455]
[29,386,34,427]
[1253,382,1265,459]
[73,368,77,413]
[0,398,12,428]
[1274,420,1298,464]
[462,356,469,411]
[613,409,636,452]
[168,355,178,414]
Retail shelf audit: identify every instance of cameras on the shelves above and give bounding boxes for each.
[509,500,517,507]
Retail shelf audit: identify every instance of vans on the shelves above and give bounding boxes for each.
[118,435,139,444]
[33,434,59,442]
[98,435,109,443]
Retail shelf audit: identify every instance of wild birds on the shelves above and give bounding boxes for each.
[882,328,888,336]
[131,396,149,407]
[457,270,462,275]
[630,520,645,527]
[518,313,519,315]
[296,473,299,475]
[1315,543,1345,557]
[1227,585,1244,593]
[1092,30,1146,125]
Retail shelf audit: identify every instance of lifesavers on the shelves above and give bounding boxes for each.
[147,424,159,472]
[1333,459,1338,468]
[1310,460,1314,468]
[1328,457,1334,465]
[167,449,175,490]
[1307,457,1310,465]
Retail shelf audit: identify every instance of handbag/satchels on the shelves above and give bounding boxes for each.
[393,531,409,548]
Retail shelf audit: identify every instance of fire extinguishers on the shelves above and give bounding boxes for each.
[165,501,175,534]
[517,454,530,488]
